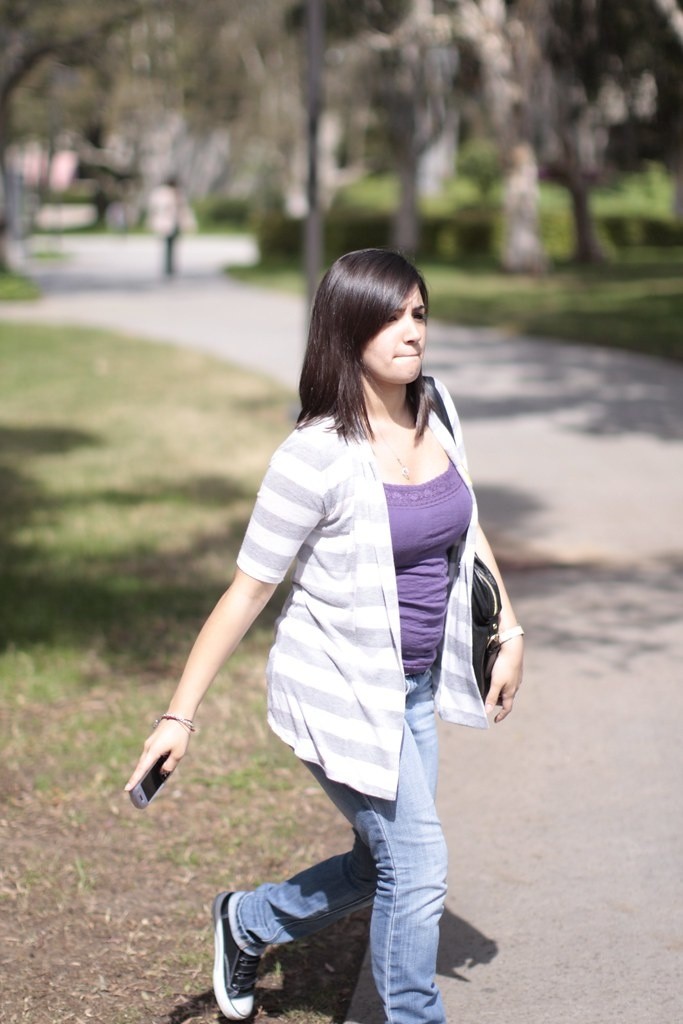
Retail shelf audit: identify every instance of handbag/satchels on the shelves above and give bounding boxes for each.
[423,377,503,706]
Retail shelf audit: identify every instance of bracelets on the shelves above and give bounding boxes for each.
[498,626,525,645]
[152,715,195,733]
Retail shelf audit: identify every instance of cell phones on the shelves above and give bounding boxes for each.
[129,755,172,809]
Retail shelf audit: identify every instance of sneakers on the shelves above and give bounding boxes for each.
[212,890,260,1020]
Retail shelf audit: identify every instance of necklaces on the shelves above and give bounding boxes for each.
[380,430,409,476]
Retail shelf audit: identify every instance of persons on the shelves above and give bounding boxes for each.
[124,248,526,1024]
